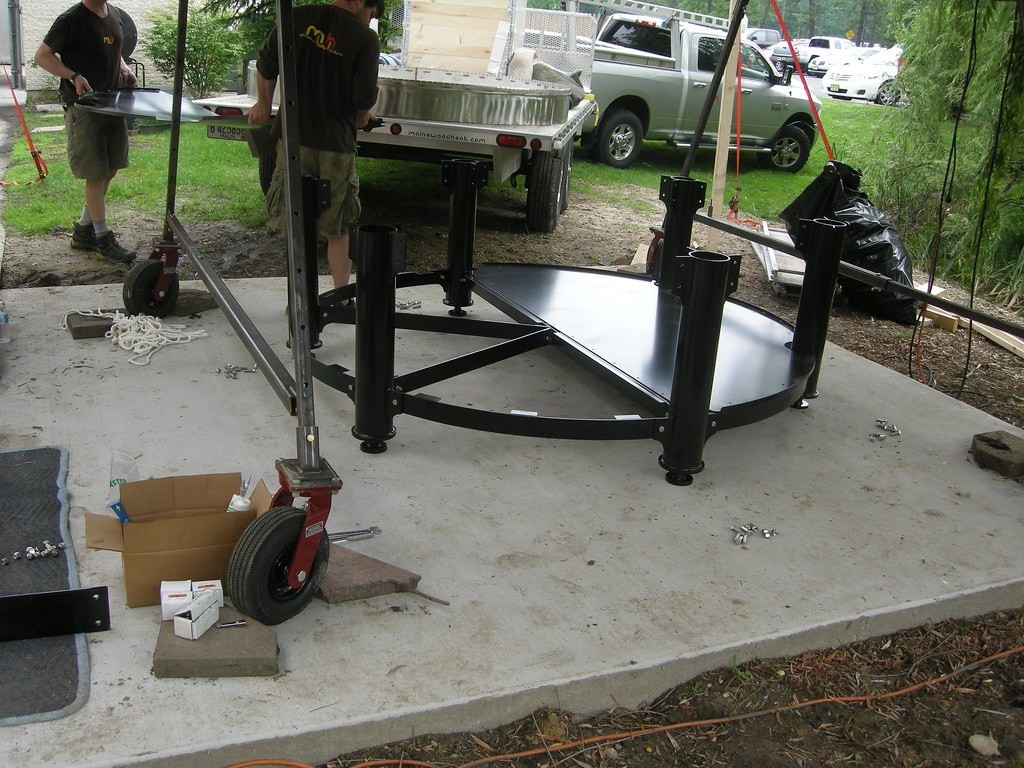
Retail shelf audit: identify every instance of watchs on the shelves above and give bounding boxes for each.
[69,71,82,85]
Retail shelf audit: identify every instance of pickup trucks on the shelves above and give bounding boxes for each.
[740,27,780,48]
[510,11,821,173]
[772,36,856,69]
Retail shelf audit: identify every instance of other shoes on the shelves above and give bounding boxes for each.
[333,298,356,308]
[70,221,98,252]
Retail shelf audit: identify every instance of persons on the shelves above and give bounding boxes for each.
[248,0,386,308]
[34,1,142,264]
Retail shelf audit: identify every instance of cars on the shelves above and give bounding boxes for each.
[808,47,887,77]
[821,43,905,107]
[748,39,810,72]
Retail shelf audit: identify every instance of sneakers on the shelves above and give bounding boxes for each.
[95,231,137,264]
